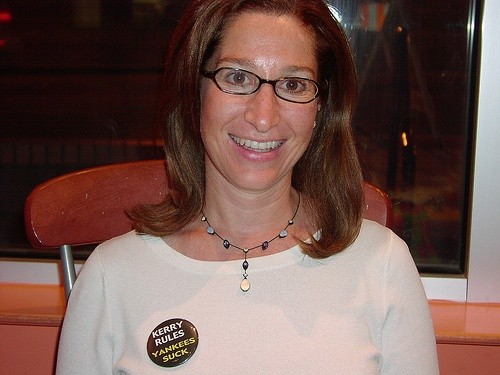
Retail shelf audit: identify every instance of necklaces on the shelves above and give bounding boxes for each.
[201,192,300,292]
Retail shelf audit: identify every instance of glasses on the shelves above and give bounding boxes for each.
[200,66,321,104]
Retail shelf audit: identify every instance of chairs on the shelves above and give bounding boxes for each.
[25,160,393,307]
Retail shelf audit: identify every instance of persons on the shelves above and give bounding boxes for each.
[55,0,441,375]
